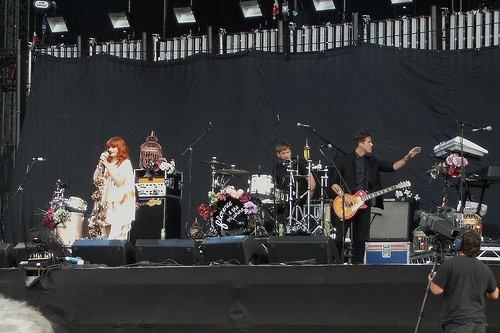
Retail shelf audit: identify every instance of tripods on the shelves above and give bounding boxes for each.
[277,155,335,237]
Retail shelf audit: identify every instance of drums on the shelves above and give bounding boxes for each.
[213,195,265,235]
[261,188,289,205]
[67,197,88,212]
[56,212,88,245]
[248,174,274,199]
[318,203,352,244]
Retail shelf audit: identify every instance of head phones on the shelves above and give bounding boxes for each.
[453,230,472,251]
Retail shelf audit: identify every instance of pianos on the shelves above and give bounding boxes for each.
[432,136,500,216]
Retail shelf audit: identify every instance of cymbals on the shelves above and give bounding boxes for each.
[200,160,228,166]
[208,169,247,175]
[286,174,308,177]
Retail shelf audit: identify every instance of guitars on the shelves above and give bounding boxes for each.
[333,179,411,219]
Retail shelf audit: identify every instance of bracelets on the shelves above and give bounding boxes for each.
[404,158,408,162]
[96,165,102,170]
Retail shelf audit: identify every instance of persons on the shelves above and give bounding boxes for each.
[328,130,422,265]
[93,137,136,241]
[271,143,316,236]
[0,294,56,333]
[427,229,500,333]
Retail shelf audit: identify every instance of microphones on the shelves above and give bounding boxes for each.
[238,222,244,225]
[297,123,310,128]
[102,164,105,178]
[321,144,333,148]
[210,122,213,129]
[277,114,280,121]
[32,157,45,161]
[472,126,493,131]
[372,212,382,216]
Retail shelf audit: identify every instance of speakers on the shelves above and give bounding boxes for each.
[129,198,182,245]
[72,234,341,268]
[367,200,410,242]
[0,243,18,268]
